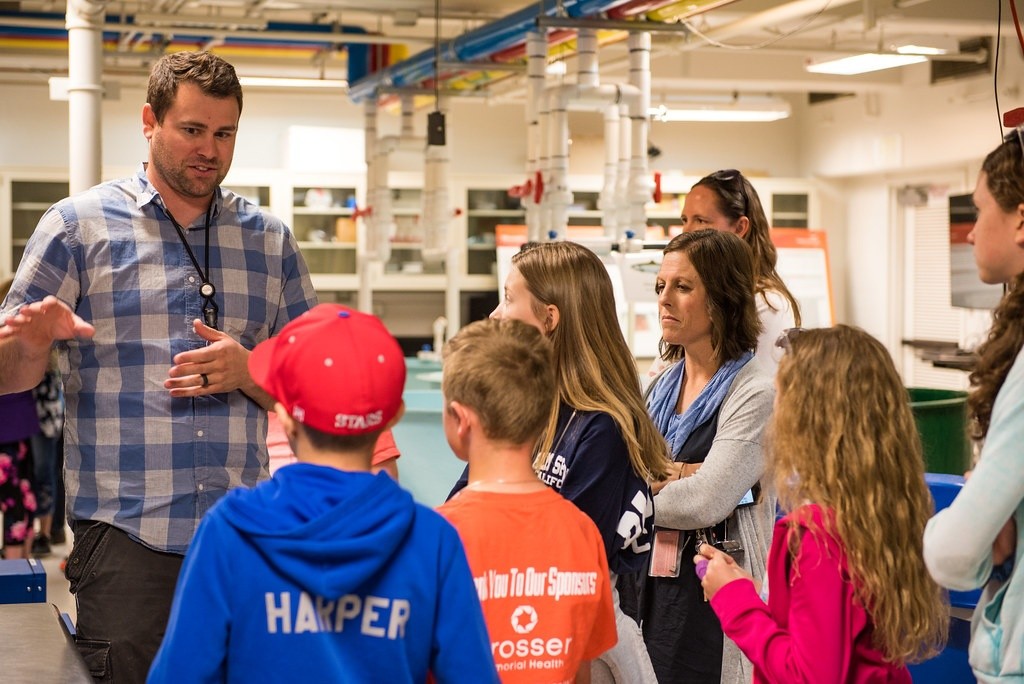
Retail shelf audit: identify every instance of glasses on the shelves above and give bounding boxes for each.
[709,169,748,217]
[1003,127,1024,156]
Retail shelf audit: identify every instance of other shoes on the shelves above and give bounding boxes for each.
[31,540,49,554]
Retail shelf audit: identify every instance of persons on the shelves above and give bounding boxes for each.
[642,229,784,684]
[445,241,671,684]
[693,325,951,684]
[923,125,1024,684]
[265,410,401,484]
[431,319,620,684]
[0,49,319,684]
[144,303,502,684]
[647,169,803,356]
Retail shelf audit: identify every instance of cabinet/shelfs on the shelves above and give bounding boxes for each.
[0,171,819,337]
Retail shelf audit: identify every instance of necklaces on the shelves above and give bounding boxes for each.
[164,207,219,329]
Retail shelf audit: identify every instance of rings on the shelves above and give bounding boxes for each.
[201,374,208,386]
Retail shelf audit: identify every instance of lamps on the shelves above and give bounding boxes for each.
[651,95,791,122]
[422,0,451,164]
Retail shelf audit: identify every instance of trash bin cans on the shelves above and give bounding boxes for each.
[907,387,973,477]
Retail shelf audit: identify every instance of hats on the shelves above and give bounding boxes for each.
[247,302,406,437]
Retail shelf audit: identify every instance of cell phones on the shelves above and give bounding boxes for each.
[736,488,756,509]
